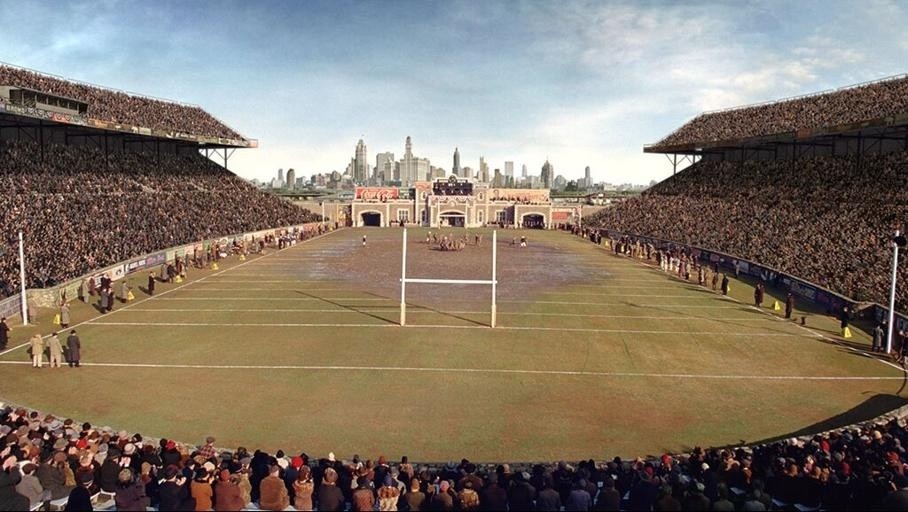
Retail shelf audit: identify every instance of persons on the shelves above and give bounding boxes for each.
[45,333,65,368]
[573,140,908,361]
[497,219,572,233]
[519,235,527,247]
[67,329,82,367]
[0,150,341,326]
[0,403,908,511]
[0,63,254,152]
[28,330,45,369]
[649,75,908,142]
[511,237,519,246]
[420,225,484,255]
[360,233,368,248]
[0,317,13,350]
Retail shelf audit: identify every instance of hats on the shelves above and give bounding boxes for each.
[2,405,899,512]
[35,330,77,338]
